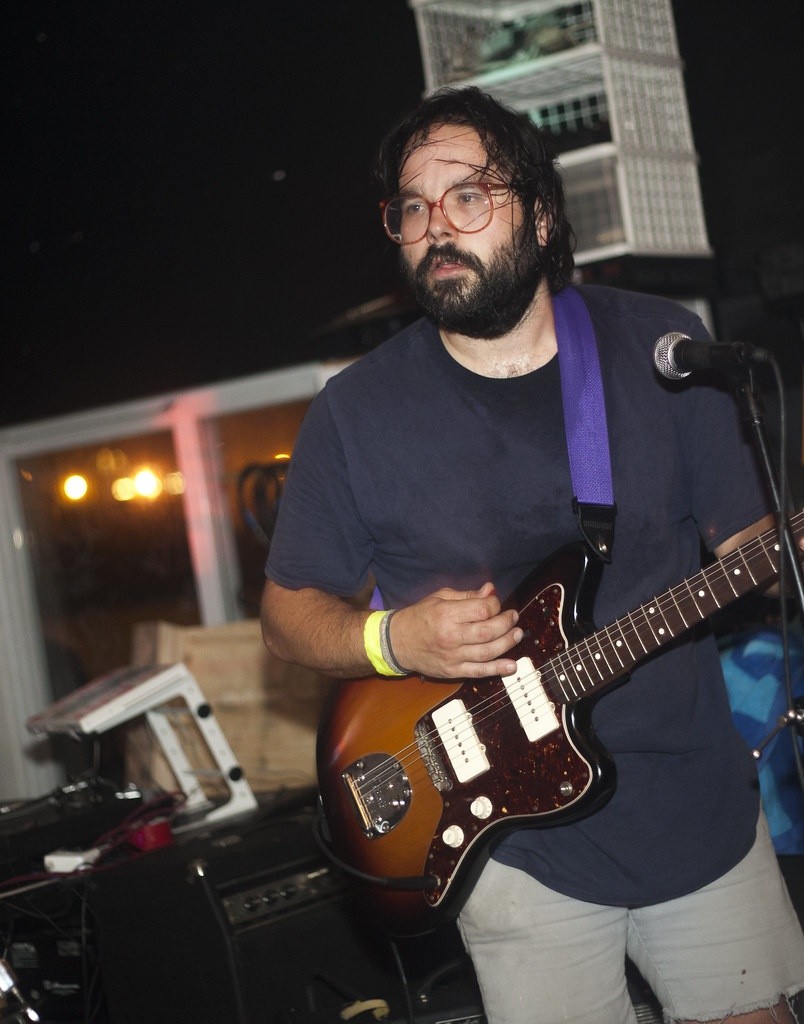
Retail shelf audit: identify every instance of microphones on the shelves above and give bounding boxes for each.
[651,332,772,379]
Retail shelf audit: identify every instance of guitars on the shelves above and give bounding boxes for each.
[316,511,804,937]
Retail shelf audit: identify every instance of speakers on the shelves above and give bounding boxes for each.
[0,773,489,1024]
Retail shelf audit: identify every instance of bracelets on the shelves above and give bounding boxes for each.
[364,610,410,676]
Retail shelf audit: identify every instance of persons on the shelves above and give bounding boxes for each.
[261,86,804,1024]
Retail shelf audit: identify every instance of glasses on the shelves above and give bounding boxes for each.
[379,183,512,245]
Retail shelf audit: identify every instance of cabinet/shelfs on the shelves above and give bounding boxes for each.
[411,0,718,267]
